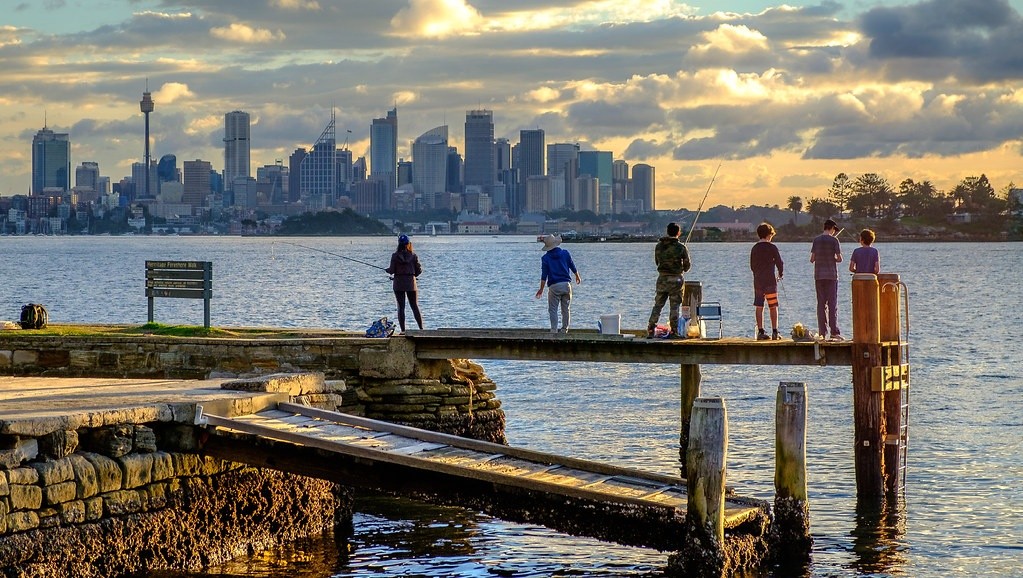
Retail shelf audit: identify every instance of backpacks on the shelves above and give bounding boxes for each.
[18,302,47,329]
[363,317,396,337]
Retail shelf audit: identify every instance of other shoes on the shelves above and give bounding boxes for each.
[670,329,680,338]
[819,335,826,341]
[830,335,844,341]
[646,332,657,339]
[550,328,567,334]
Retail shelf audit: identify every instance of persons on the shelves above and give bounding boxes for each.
[810,219,845,342]
[848,229,880,273]
[384,236,424,334]
[749,222,784,340]
[534,234,580,333]
[645,222,691,333]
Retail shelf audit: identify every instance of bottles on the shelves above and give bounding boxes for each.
[679,316,685,336]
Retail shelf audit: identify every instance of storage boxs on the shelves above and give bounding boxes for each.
[599,313,621,335]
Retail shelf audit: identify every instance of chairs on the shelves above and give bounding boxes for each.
[695,302,724,340]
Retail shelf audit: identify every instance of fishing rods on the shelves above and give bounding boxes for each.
[270,241,419,281]
[776,277,795,331]
[835,228,857,242]
[682,152,726,246]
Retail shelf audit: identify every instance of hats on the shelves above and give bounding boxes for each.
[542,233,562,251]
[398,234,412,244]
[825,220,840,230]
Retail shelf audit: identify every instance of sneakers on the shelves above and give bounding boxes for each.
[756,332,769,342]
[774,332,782,340]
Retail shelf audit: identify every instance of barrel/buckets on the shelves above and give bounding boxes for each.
[600,313,620,334]
